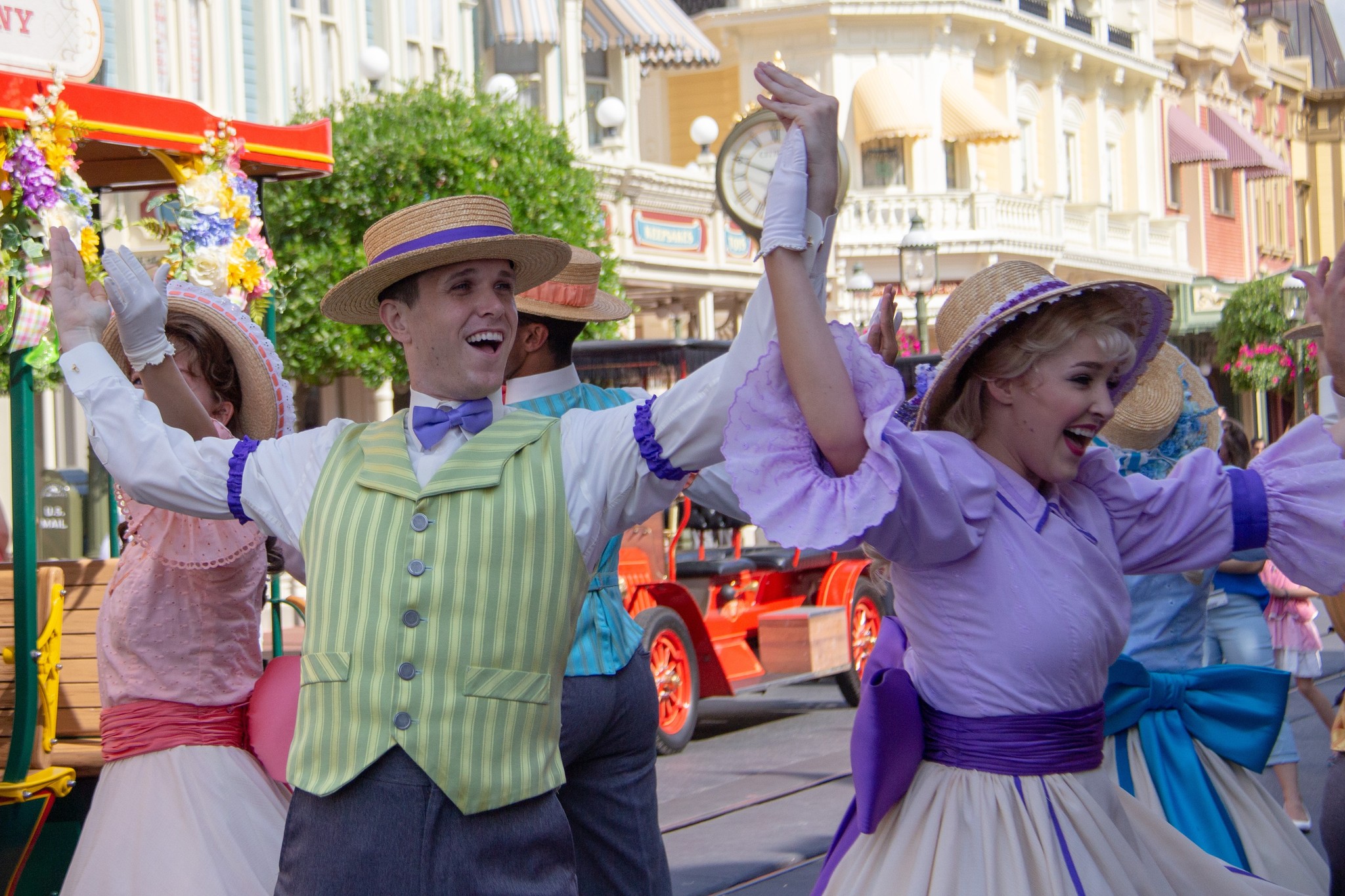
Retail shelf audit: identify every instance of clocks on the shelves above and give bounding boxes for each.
[718,52,850,246]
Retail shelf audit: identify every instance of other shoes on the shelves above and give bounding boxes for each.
[1282,801,1311,832]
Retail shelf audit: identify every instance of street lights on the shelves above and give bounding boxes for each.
[844,261,875,338]
[895,210,938,357]
[1281,261,1307,423]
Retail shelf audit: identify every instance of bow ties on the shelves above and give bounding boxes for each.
[412,396,494,449]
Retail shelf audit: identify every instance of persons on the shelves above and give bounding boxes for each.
[48,64,850,896]
[753,117,1345,896]
[500,245,753,896]
[59,245,306,896]
[1090,240,1345,896]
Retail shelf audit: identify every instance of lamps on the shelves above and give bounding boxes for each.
[358,46,390,104]
[690,115,719,173]
[594,96,628,149]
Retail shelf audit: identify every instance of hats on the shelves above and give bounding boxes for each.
[514,243,632,321]
[320,195,572,327]
[1086,339,1223,481]
[102,277,298,442]
[895,259,1174,433]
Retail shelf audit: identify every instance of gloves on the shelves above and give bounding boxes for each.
[753,118,811,262]
[101,244,177,371]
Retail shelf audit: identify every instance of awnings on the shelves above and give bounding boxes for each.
[851,67,934,145]
[1168,105,1229,167]
[941,76,1023,145]
[482,0,561,49]
[583,0,721,71]
[1207,107,1291,180]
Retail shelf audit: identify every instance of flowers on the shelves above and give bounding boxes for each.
[0,65,295,393]
[1223,341,1295,392]
